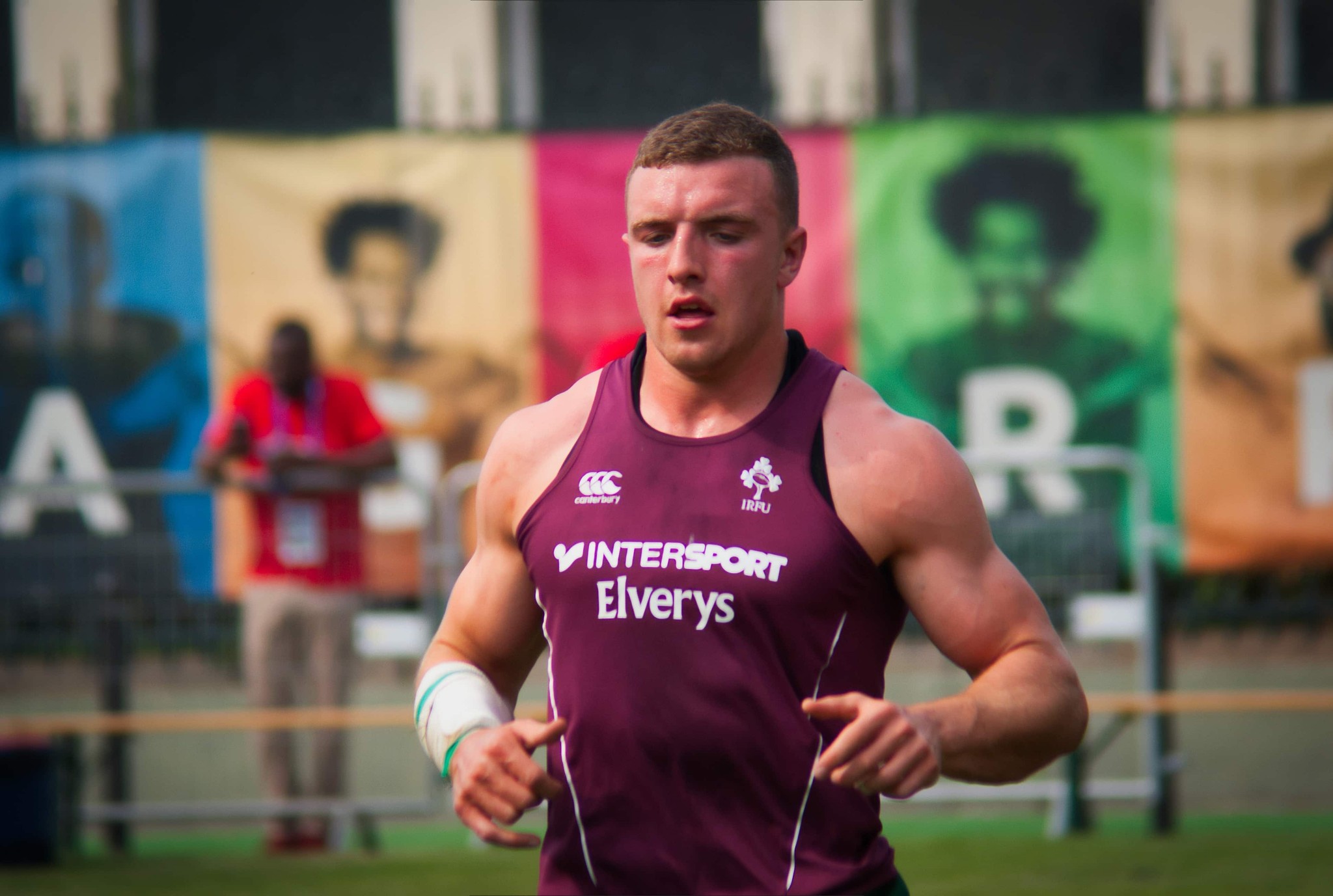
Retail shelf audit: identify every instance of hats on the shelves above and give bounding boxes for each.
[925,137,1105,273]
[319,194,445,283]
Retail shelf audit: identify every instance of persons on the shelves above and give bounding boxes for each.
[197,319,399,854]
[0,142,1333,644]
[411,104,1089,895]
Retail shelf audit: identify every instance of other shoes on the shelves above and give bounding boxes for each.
[295,816,332,854]
[264,817,294,852]
